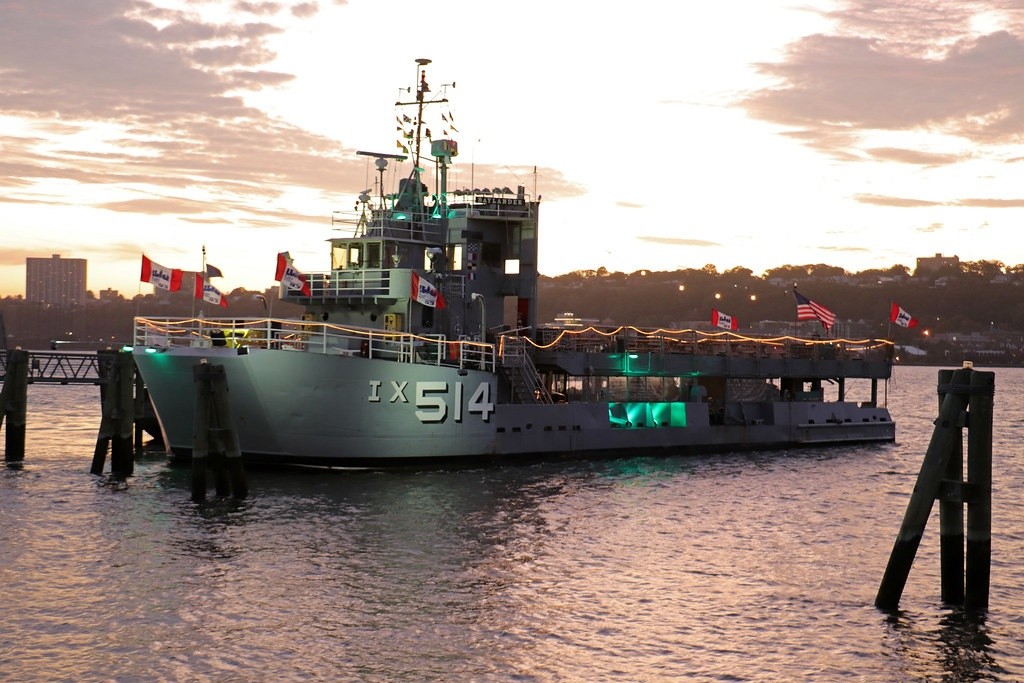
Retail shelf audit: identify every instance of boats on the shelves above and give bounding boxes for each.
[126,54,902,472]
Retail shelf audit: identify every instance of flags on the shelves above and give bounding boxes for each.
[194,273,228,309]
[274,253,312,297]
[140,254,182,292]
[207,264,223,278]
[411,270,446,310]
[711,308,738,331]
[891,301,920,329]
[793,289,835,333]
